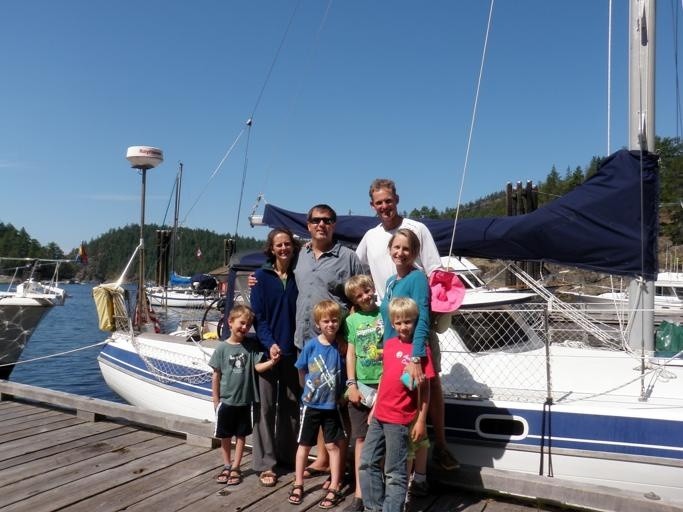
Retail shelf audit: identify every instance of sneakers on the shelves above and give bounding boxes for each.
[411,479,432,498]
[431,444,461,473]
[343,496,364,512]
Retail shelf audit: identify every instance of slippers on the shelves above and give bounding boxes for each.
[323,477,347,490]
[301,465,331,478]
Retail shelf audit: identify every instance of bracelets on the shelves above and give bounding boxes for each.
[344,378,358,386]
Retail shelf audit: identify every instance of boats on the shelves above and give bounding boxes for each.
[0,253,85,382]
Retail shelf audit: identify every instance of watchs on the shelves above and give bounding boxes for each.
[410,357,422,365]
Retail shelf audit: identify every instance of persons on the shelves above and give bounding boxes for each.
[343,274,386,512]
[250,227,300,487]
[288,299,347,505]
[380,228,433,497]
[355,177,461,471]
[358,297,439,512]
[246,202,367,480]
[207,303,283,486]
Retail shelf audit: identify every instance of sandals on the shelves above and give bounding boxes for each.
[318,488,346,509]
[258,467,277,487]
[288,482,304,505]
[217,465,232,483]
[227,467,242,485]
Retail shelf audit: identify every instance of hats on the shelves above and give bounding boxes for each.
[428,269,466,313]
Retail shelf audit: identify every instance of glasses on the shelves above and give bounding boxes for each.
[309,217,333,225]
